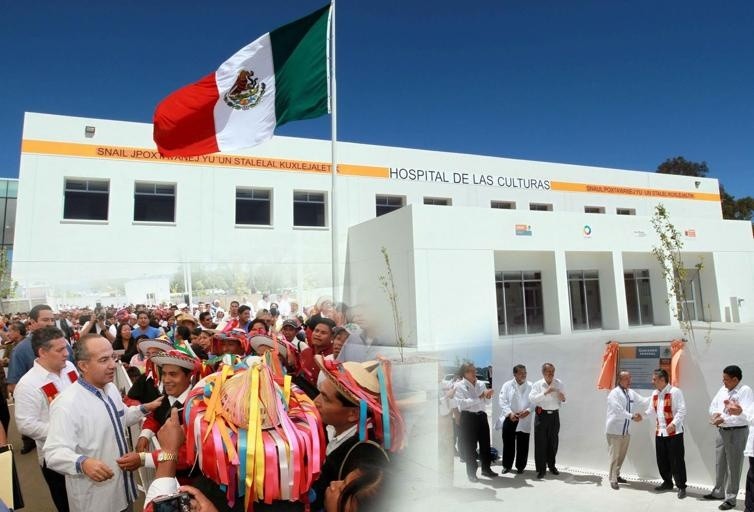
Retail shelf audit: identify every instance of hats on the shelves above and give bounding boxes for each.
[183,362,320,465]
[138,333,196,371]
[216,328,298,359]
[332,322,360,335]
[283,320,297,328]
[313,353,381,407]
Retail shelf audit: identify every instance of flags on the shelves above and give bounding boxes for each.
[154,2,330,158]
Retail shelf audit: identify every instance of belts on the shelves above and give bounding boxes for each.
[723,425,746,430]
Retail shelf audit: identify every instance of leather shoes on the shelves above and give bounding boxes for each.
[655,483,673,490]
[719,501,736,510]
[703,494,725,499]
[611,477,627,490]
[678,488,685,498]
[469,466,559,482]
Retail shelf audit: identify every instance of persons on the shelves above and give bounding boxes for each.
[1,295,405,511]
[439,363,753,510]
